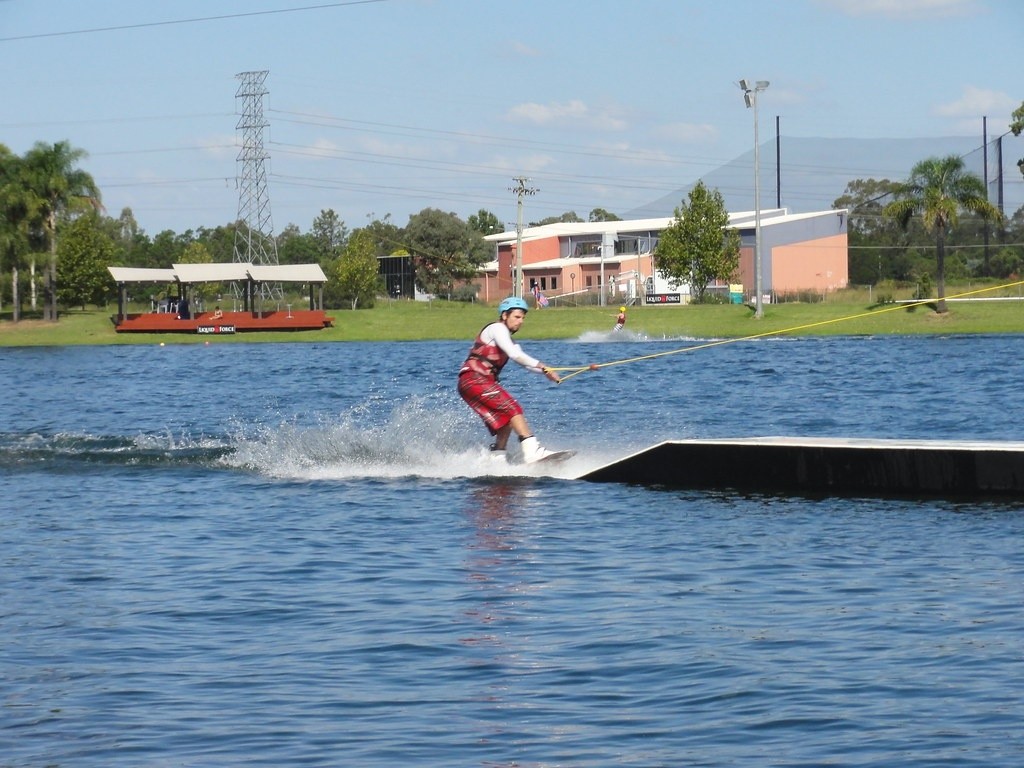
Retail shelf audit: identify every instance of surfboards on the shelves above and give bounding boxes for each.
[531,287,549,308]
[518,450,578,474]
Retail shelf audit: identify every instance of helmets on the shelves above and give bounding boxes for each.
[499,297,528,317]
[620,306,626,312]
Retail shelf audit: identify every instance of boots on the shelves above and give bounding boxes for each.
[522,437,556,466]
[489,449,510,466]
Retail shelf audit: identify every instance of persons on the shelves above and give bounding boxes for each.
[458,298,560,466]
[612,307,626,332]
[209,306,223,320]
[534,282,542,310]
